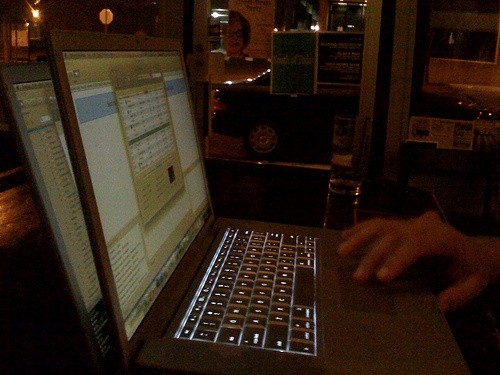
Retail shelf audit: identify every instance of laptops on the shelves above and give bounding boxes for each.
[47,31,468,371]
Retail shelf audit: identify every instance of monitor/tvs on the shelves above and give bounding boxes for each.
[1,60,122,372]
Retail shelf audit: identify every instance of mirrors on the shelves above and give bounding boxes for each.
[204,1,379,172]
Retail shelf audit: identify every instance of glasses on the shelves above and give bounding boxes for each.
[224,29,244,39]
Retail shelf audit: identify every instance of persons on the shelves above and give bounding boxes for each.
[214,9,270,81]
[336,209,500,311]
